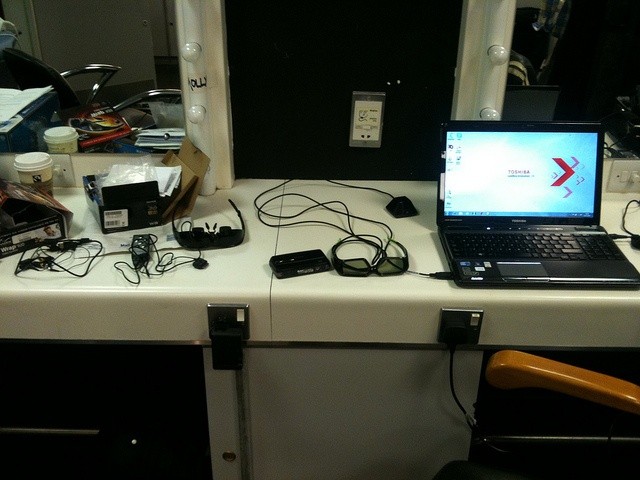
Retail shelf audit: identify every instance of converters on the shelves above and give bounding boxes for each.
[211,328,243,370]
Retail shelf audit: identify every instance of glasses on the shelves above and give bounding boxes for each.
[172,198,245,250]
[68,118,124,132]
[330,237,410,277]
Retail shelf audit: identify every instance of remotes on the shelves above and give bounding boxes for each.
[132,234,150,269]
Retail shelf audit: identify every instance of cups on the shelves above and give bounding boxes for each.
[13,151,53,204]
[43,126,80,153]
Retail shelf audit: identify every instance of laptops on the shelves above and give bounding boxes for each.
[437,120,640,291]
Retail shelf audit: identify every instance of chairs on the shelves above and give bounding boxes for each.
[431,350,640,480]
[2,48,181,110]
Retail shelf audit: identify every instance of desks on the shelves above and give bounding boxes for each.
[0,153,640,480]
